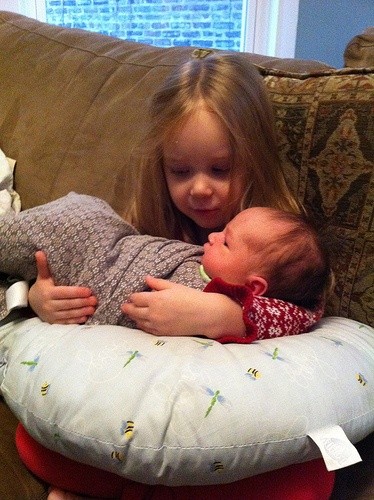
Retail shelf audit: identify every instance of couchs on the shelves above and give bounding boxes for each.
[0,8,374,500]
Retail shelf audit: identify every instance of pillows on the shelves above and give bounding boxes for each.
[192,48,374,328]
[0,315,374,488]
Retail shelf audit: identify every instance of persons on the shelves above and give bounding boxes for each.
[15,50,336,500]
[0,191,331,331]
